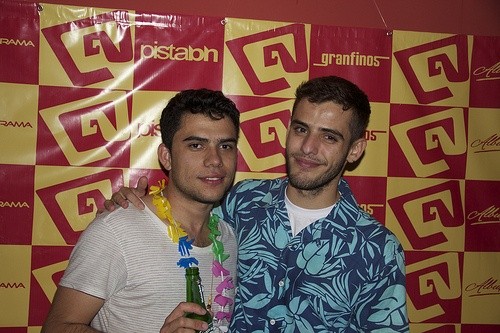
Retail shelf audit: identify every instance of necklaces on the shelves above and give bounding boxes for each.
[149,179,235,332]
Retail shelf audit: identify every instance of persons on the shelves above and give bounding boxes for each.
[96,76,410,333]
[40,88,241,333]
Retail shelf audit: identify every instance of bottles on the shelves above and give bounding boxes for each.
[185,267,213,333]
